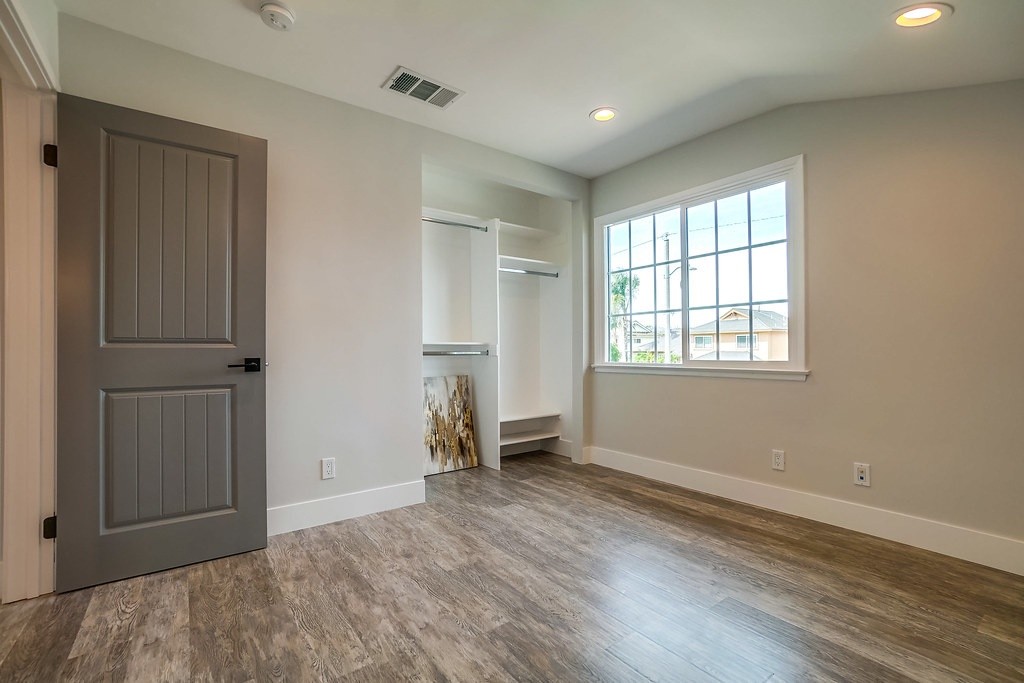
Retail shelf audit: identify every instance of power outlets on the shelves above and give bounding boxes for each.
[321,457,335,480]
[771,449,784,471]
